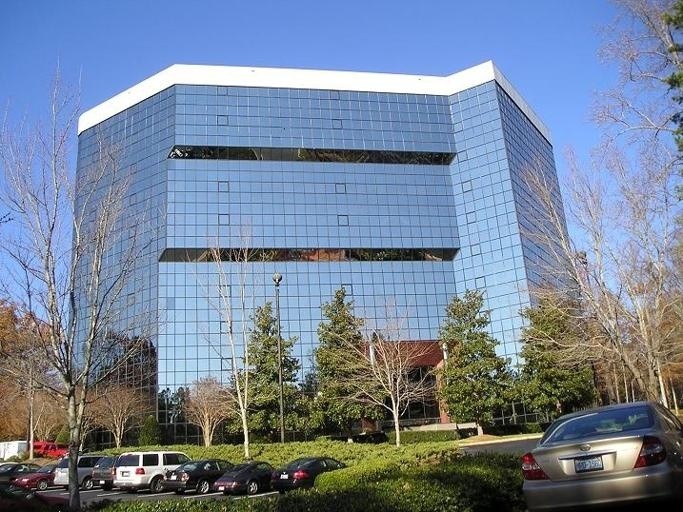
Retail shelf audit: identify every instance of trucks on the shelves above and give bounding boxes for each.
[33,441,68,459]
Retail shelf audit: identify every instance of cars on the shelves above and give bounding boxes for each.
[0,463,44,484]
[12,460,61,491]
[270,457,347,494]
[519,401,683,511]
[0,480,69,512]
[212,461,277,495]
[164,459,234,494]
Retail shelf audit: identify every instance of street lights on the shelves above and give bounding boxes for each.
[573,248,600,320]
[272,273,285,446]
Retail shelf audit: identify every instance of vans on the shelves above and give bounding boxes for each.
[92,455,120,491]
[112,451,194,495]
[54,456,105,491]
